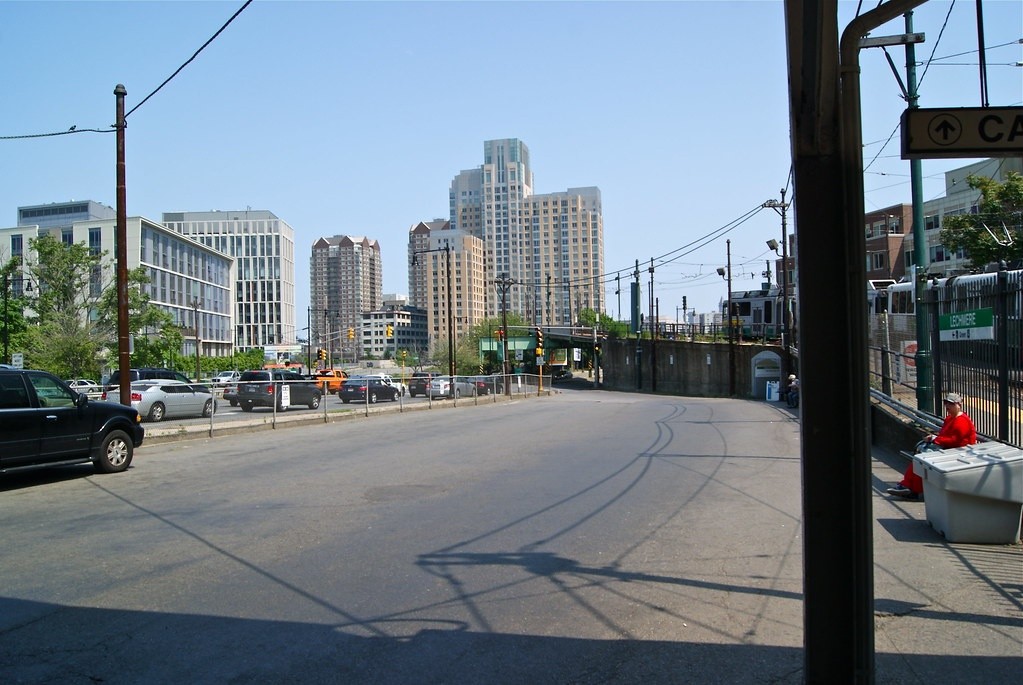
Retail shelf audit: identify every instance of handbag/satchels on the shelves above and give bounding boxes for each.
[916,435,942,452]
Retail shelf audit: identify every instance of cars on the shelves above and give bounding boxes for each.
[425,374,477,397]
[0,368,147,477]
[211,371,240,388]
[224,377,240,408]
[102,368,211,395]
[57,378,98,398]
[368,375,406,397]
[234,369,322,413]
[464,374,503,396]
[338,375,399,403]
[409,371,444,398]
[101,379,217,420]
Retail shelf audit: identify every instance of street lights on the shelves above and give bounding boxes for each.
[306,307,328,377]
[324,309,340,370]
[3,273,33,364]
[169,318,187,370]
[413,245,456,399]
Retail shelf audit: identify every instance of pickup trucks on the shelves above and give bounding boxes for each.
[309,370,348,393]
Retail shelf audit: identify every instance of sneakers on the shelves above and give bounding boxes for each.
[887,486,918,498]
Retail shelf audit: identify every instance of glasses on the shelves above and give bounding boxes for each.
[944,401,954,404]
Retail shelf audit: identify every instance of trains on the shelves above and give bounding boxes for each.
[719,262,1023,374]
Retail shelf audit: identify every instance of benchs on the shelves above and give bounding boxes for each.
[899,450,915,461]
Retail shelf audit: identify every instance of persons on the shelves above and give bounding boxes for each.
[887,392,976,497]
[785,370,800,409]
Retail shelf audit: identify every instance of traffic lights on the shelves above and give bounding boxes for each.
[349,328,355,339]
[387,325,393,338]
[322,349,326,360]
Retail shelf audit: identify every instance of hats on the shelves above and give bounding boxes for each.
[944,393,961,403]
[788,375,795,379]
